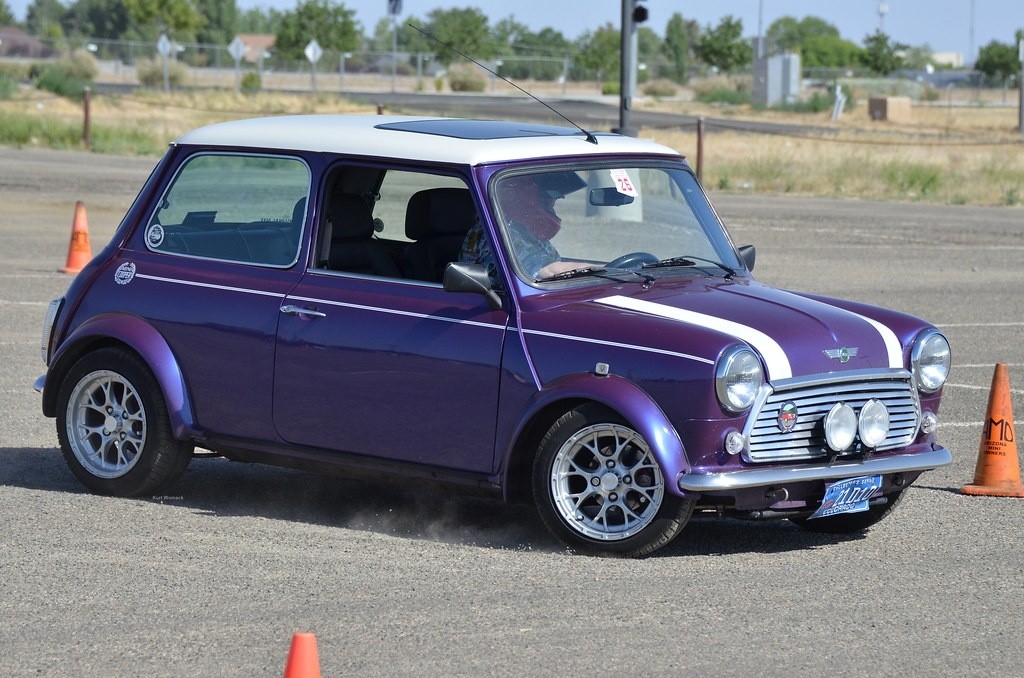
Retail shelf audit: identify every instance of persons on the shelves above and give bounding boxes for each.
[461,170,607,282]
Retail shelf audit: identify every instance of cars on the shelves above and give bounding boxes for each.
[33,112,952,560]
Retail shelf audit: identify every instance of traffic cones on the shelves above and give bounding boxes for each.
[959,363,1024,497]
[283,632,322,678]
[55,200,92,275]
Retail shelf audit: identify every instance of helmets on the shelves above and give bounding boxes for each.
[498,171,587,241]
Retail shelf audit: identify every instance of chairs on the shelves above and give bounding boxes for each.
[287,189,377,275]
[404,187,476,284]
